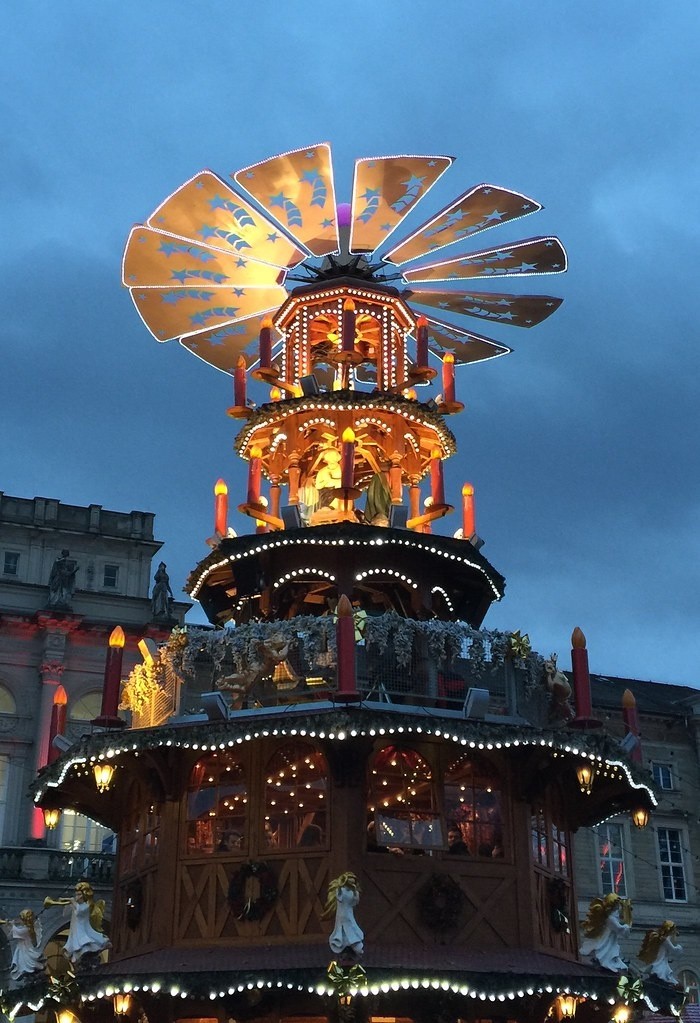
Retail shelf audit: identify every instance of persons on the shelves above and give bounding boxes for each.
[217,820,324,854]
[316,450,342,510]
[60,882,113,962]
[322,872,364,956]
[637,921,683,986]
[10,909,46,981]
[47,548,79,611]
[579,893,631,973]
[366,820,503,858]
[151,560,174,617]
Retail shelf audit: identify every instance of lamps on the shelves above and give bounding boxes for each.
[53,1007,82,1023]
[631,807,650,830]
[339,995,352,1010]
[113,993,133,1016]
[575,764,597,795]
[556,994,578,1021]
[91,763,116,793]
[611,1006,633,1023]
[41,808,63,830]
[40,297,644,769]
